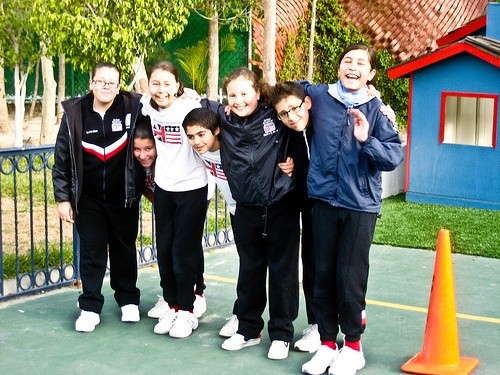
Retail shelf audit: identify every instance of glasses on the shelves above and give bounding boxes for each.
[92,79,119,89]
[277,95,306,121]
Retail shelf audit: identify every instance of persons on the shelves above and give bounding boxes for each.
[200,68,380,359]
[270,81,396,352]
[182,108,294,337]
[132,62,207,338]
[225,44,403,375]
[52,63,201,331]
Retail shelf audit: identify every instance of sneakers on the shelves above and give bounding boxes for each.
[120,304,140,322]
[193,293,207,318]
[74,309,100,332]
[267,340,290,360]
[218,315,239,338]
[328,335,369,375]
[153,308,178,334]
[221,330,263,351]
[169,310,199,338]
[147,295,171,318]
[293,324,321,353]
[302,341,338,375]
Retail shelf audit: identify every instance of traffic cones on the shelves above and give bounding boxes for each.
[400,228,479,375]
